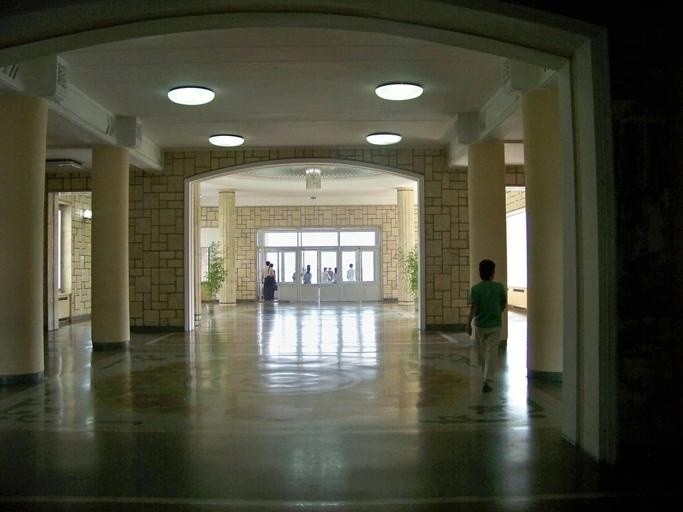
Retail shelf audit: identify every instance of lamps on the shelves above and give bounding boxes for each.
[209,134,245,148]
[167,85,215,107]
[364,132,402,147]
[374,80,424,102]
[305,169,322,200]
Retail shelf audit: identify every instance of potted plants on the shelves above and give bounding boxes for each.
[202,240,228,312]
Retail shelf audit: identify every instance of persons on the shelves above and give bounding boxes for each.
[326,267,333,281]
[464,258,509,394]
[261,261,273,301]
[330,268,337,283]
[302,264,311,285]
[321,267,331,284]
[346,263,354,281]
[267,264,275,300]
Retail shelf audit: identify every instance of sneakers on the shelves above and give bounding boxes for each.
[481,386,493,393]
